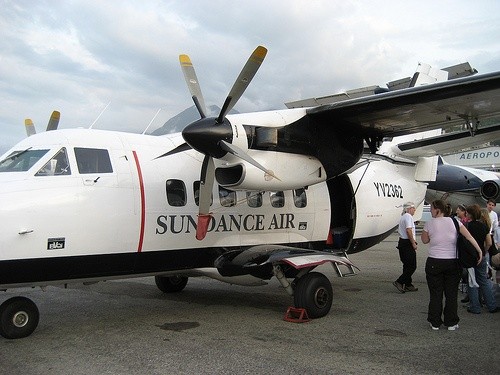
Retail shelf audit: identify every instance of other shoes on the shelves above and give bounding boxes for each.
[461,295,469,302]
[484,306,496,313]
[430,322,439,330]
[448,324,459,330]
[405,284,418,291]
[393,281,405,293]
[467,306,480,314]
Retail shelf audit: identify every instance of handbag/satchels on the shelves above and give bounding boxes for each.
[451,216,480,268]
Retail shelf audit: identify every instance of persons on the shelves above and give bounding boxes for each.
[393,201,418,294]
[420,200,500,330]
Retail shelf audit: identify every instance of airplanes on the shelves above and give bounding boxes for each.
[0,44,500,340]
